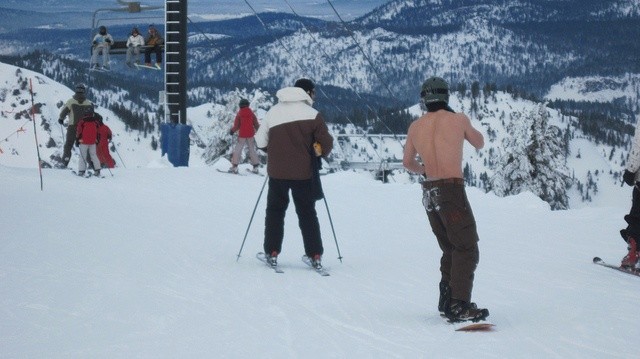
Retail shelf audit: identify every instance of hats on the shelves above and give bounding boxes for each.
[294,79,314,92]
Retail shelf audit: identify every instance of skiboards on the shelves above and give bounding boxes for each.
[139,63,161,70]
[217,168,264,178]
[72,168,105,178]
[88,66,109,73]
[257,252,330,277]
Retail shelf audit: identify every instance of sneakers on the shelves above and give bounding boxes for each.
[621,235,640,267]
[443,286,489,321]
[252,164,258,174]
[438,282,477,312]
[308,253,322,269]
[266,250,277,266]
[228,164,238,173]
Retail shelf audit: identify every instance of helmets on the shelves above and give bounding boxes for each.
[239,99,249,107]
[76,83,85,94]
[420,76,448,110]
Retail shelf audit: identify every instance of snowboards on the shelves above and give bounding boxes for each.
[441,313,494,331]
[593,257,640,276]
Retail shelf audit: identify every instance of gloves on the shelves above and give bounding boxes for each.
[58,119,63,124]
[623,170,635,186]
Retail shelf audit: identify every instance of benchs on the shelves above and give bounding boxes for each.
[91,42,165,54]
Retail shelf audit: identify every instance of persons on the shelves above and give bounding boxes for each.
[125,27,144,68]
[75,106,102,177]
[253,78,334,270]
[401,77,490,323]
[90,23,115,73]
[144,26,165,69]
[619,116,639,268]
[54,84,94,169]
[228,99,260,174]
[95,113,116,169]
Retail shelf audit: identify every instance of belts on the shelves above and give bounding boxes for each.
[418,178,464,188]
